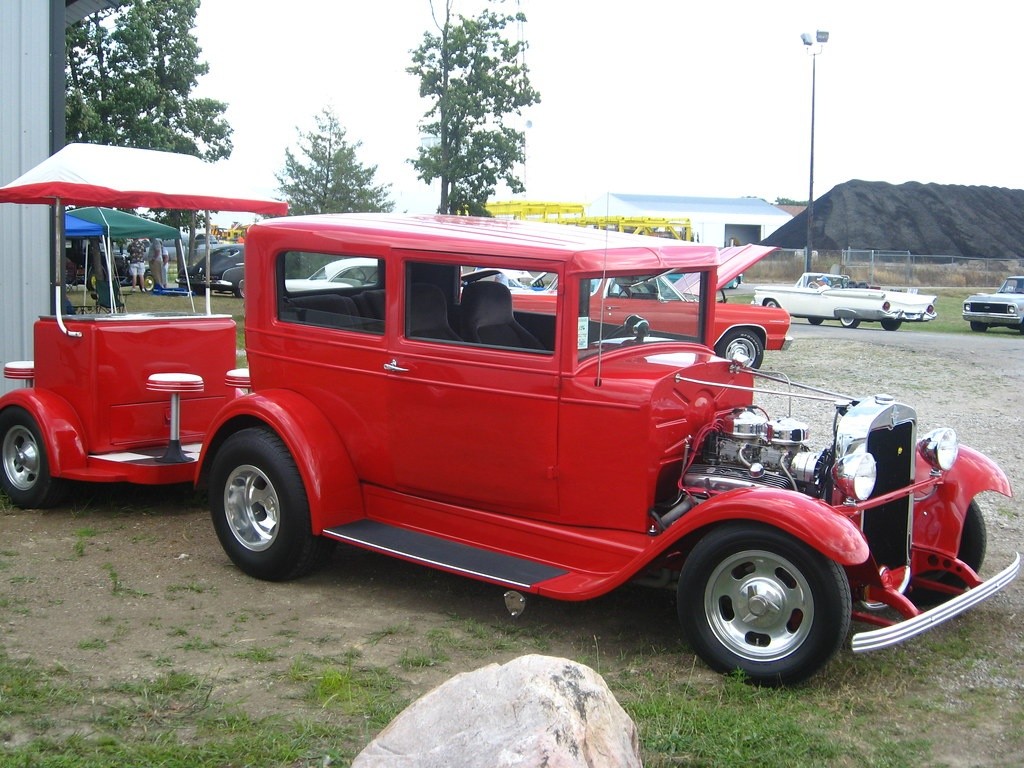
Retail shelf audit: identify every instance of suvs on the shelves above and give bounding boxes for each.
[187,212,1022,688]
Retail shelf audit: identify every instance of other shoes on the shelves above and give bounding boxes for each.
[142,288,146,292]
[129,289,136,292]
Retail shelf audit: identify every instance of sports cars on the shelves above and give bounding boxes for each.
[750,272,938,331]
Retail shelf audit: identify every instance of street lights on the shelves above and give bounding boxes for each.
[799,28,830,274]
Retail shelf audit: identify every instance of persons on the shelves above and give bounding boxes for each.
[816,276,829,287]
[127,239,145,293]
[65,237,169,288]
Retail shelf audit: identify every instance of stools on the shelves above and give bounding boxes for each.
[146,373,199,464]
[3,361,34,388]
[225,367,252,395]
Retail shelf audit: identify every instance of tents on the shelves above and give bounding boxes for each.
[64,215,116,315]
[66,206,197,316]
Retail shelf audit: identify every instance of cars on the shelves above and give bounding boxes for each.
[963,275,1024,336]
[172,242,249,300]
[457,243,793,377]
[62,228,220,291]
[302,254,381,292]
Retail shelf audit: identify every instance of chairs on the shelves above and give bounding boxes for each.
[66,263,79,293]
[91,281,132,314]
[290,279,549,351]
